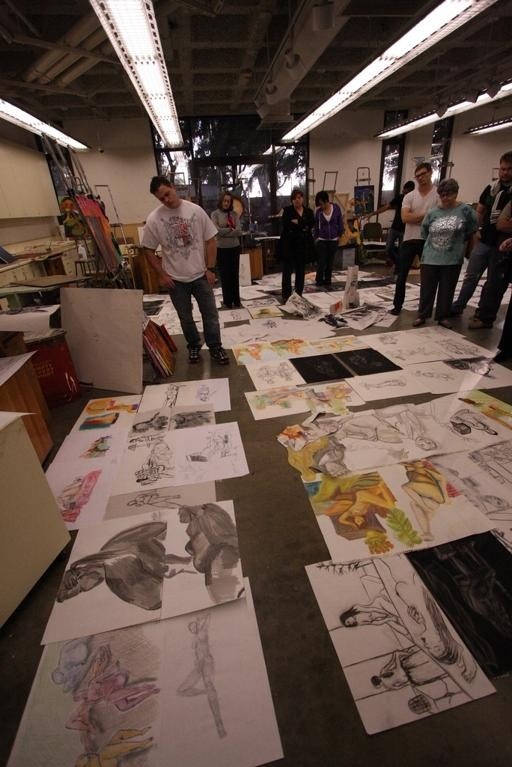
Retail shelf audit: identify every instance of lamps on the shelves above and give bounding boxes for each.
[0,88,91,154]
[251,0,511,144]
[88,0,185,149]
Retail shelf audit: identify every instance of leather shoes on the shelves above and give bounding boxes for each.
[391,307,400,314]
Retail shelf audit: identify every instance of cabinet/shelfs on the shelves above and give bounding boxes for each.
[1,238,99,631]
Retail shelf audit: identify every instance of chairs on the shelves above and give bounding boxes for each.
[360,222,390,266]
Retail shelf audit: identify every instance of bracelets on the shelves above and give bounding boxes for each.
[207,267,216,272]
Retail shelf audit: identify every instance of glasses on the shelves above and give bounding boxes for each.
[414,171,428,179]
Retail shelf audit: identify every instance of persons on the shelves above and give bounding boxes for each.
[409,177,480,329]
[336,483,394,535]
[371,646,416,692]
[466,198,512,329]
[58,197,80,224]
[451,151,511,315]
[377,409,439,452]
[282,407,352,480]
[176,612,229,740]
[376,555,478,684]
[209,191,245,310]
[362,181,415,282]
[139,175,230,363]
[400,459,451,544]
[341,596,413,638]
[313,190,346,292]
[277,187,315,305]
[387,161,441,316]
[41,634,161,767]
[499,237,512,364]
[456,410,499,438]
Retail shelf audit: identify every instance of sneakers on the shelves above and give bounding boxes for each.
[210,345,229,363]
[234,301,240,307]
[469,319,493,329]
[189,346,201,362]
[493,351,512,362]
[438,319,452,328]
[225,302,233,309]
[413,318,425,326]
